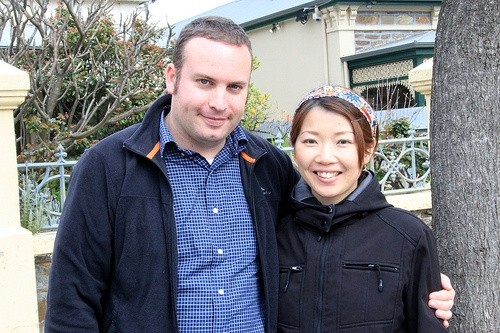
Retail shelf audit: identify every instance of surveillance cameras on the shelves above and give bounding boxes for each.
[313,13,321,22]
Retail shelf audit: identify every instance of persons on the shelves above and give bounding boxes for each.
[45,16,456,333]
